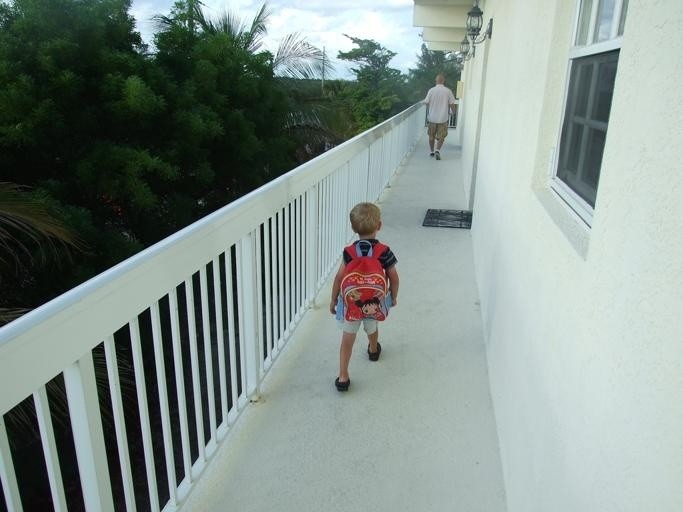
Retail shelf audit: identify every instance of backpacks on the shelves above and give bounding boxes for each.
[339,239,388,322]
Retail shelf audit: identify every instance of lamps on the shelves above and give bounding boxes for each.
[457,37,479,59]
[466,2,492,60]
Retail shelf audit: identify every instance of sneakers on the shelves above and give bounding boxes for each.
[430,152,434,157]
[434,151,440,160]
[367,342,380,360]
[334,377,349,391]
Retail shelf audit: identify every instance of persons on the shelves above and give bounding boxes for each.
[328,202,399,392]
[425,75,455,160]
